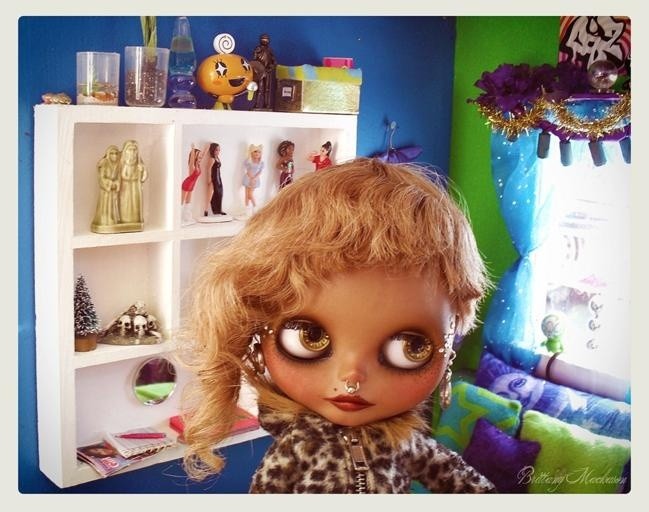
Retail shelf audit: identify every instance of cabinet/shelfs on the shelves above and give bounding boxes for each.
[34,103,358,490]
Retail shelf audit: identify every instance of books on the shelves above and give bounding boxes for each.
[103,425,177,459]
[169,406,260,438]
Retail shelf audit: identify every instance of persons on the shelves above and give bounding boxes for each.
[121,139,148,224]
[182,143,210,207]
[253,33,278,108]
[307,140,334,174]
[183,158,497,494]
[242,143,265,208]
[276,140,297,193]
[204,143,227,217]
[92,144,121,225]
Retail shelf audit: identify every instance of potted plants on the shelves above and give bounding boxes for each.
[123,15,172,108]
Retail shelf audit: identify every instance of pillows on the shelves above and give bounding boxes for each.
[431,348,630,494]
